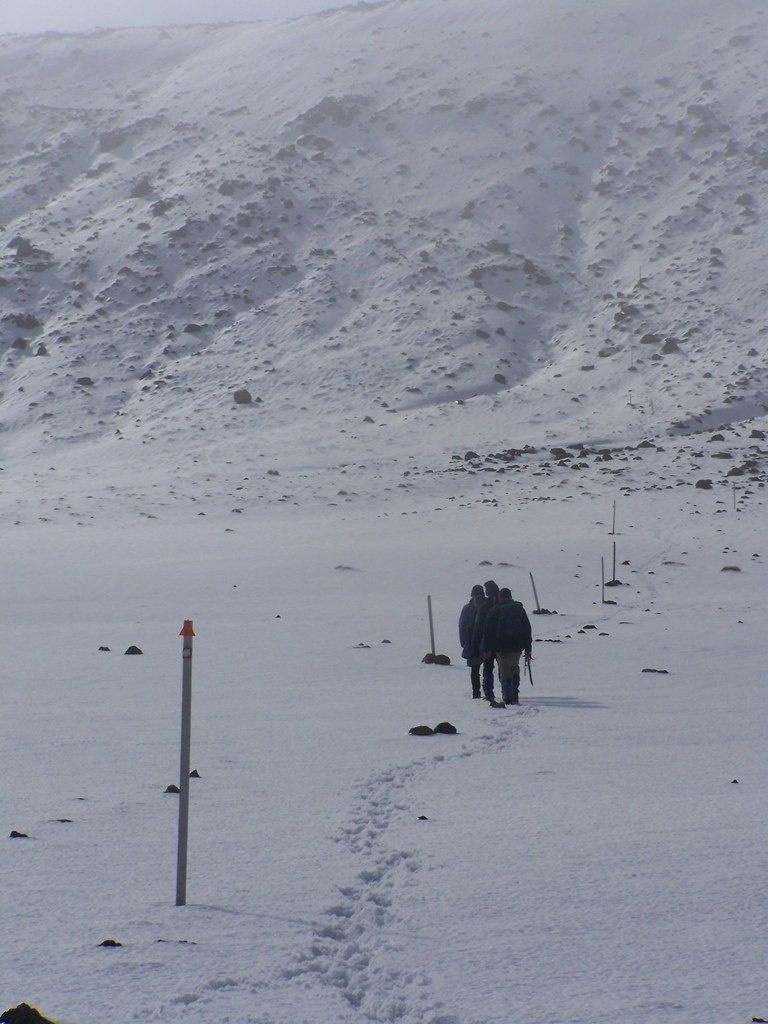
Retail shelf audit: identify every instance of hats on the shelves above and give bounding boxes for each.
[471,585,485,596]
[484,581,499,592]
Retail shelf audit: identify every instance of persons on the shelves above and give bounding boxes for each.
[470,580,502,701]
[458,585,489,698]
[484,587,533,705]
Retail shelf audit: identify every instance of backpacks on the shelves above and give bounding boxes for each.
[493,604,524,637]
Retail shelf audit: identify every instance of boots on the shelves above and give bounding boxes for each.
[502,674,520,704]
[483,673,496,702]
[471,673,481,698]
